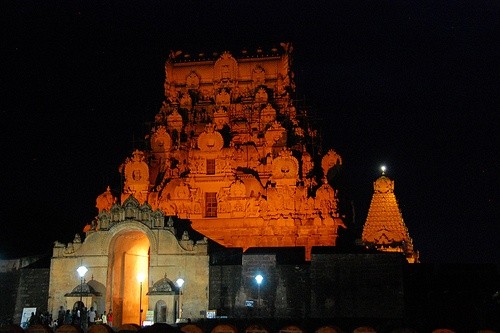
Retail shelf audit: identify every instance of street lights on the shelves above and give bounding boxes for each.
[175,278,185,319]
[76,265,88,327]
[254,275,264,317]
[137,272,145,326]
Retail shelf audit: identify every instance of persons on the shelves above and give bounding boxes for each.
[58,306,80,327]
[82,307,98,328]
[28,312,56,326]
[102,309,113,327]
